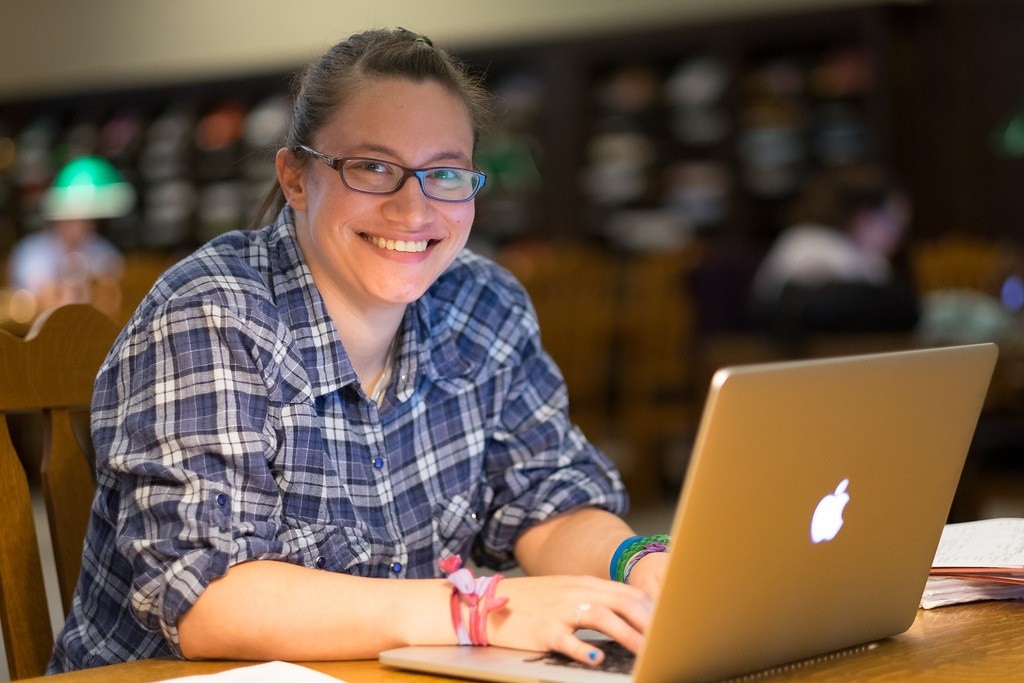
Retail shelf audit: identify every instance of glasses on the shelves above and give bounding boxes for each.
[296,143,484,202]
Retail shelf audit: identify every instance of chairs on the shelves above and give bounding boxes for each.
[0,299,122,677]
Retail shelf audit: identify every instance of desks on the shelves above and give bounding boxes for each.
[23,602,1024,683]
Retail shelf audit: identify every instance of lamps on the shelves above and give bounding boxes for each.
[36,151,136,218]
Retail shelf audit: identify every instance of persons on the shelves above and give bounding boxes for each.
[754,166,913,335]
[13,217,123,290]
[53,28,669,666]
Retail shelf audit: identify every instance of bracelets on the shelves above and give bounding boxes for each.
[609,534,671,583]
[439,555,507,647]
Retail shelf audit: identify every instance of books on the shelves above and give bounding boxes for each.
[921,576,1024,610]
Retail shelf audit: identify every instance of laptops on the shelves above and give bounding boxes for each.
[378,342,998,683]
